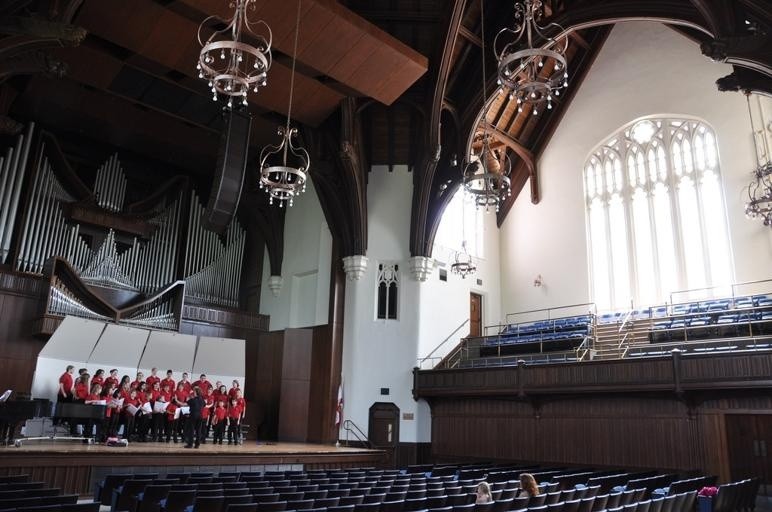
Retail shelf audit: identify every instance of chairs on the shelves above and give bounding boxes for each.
[0,463,760,512]
[460,296,772,366]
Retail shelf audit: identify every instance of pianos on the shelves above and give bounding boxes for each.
[0,391,53,445]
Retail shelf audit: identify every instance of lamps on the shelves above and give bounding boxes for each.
[740,93,772,227]
[197,0,273,107]
[259,0,311,207]
[493,0,568,116]
[451,217,476,279]
[462,0,512,214]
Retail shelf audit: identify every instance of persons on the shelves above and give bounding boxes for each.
[518,473,539,497]
[53,365,247,445]
[175,388,215,448]
[475,481,493,504]
